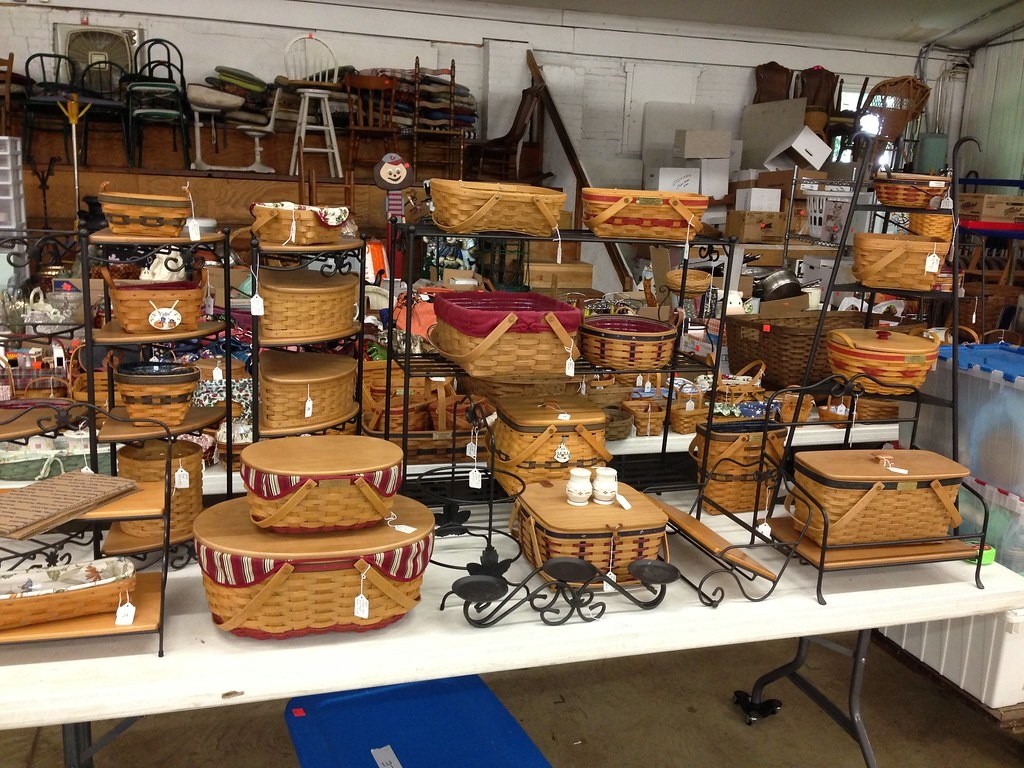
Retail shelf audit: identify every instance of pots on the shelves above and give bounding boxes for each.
[216,243,243,267]
[755,270,822,302]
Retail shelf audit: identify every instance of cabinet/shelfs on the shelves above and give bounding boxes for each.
[0,402,178,658]
[77,213,232,572]
[748,131,990,607]
[383,215,739,510]
[250,225,369,445]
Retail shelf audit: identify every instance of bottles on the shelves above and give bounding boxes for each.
[565,468,593,507]
[25,351,32,368]
[591,467,619,506]
[17,350,25,369]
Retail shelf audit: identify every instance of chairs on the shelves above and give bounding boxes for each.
[115,37,193,172]
[827,72,932,176]
[237,88,282,172]
[0,52,25,137]
[344,69,402,183]
[747,61,845,168]
[21,51,79,170]
[73,61,132,165]
[408,54,465,180]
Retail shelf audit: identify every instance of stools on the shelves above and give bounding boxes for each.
[289,88,345,181]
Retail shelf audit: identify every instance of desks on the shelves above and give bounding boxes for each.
[1,402,899,502]
[1,504,1024,766]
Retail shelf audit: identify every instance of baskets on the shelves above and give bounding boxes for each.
[100,266,208,332]
[723,309,928,396]
[73,370,126,428]
[832,397,899,423]
[910,211,955,248]
[764,390,814,425]
[426,396,488,432]
[431,179,565,235]
[875,170,951,207]
[582,186,709,240]
[793,449,973,544]
[241,435,403,532]
[252,399,359,442]
[0,366,67,399]
[100,183,190,236]
[624,400,665,437]
[252,201,351,243]
[509,476,671,587]
[362,359,456,437]
[580,314,679,371]
[483,393,613,495]
[257,351,357,425]
[933,281,1020,336]
[601,404,634,440]
[695,420,788,513]
[670,382,709,432]
[112,363,200,424]
[119,442,205,536]
[0,555,135,630]
[719,384,767,403]
[829,324,941,394]
[820,403,857,428]
[850,232,948,288]
[191,491,437,639]
[710,403,776,422]
[425,290,580,376]
[258,268,361,334]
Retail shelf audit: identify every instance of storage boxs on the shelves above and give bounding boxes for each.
[897,337,1022,501]
[282,674,552,768]
[874,609,1023,708]
[946,476,1024,576]
